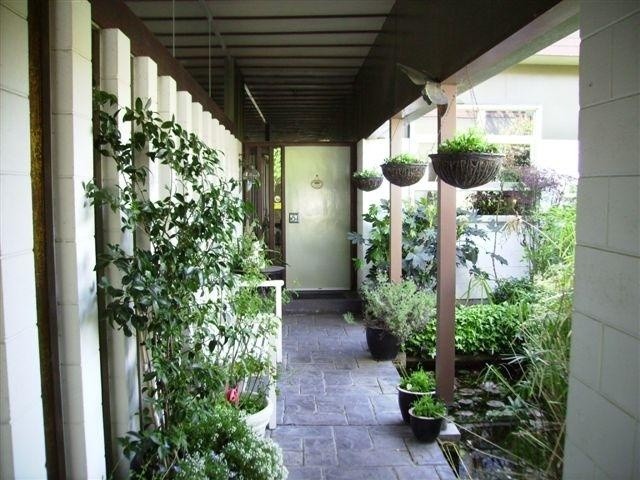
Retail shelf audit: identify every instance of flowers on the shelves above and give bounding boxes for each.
[168,386,289,480]
[472,165,559,204]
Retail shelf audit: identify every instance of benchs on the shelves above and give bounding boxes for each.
[182,274,285,430]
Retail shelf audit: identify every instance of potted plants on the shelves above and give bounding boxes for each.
[344,266,448,442]
[215,235,301,440]
[353,129,505,190]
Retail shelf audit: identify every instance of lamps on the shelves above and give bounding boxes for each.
[240,152,259,185]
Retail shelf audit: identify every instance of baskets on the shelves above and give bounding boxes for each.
[378,162,427,187]
[428,152,506,189]
[352,174,383,192]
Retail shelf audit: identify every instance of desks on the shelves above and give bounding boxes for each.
[231,266,285,296]
